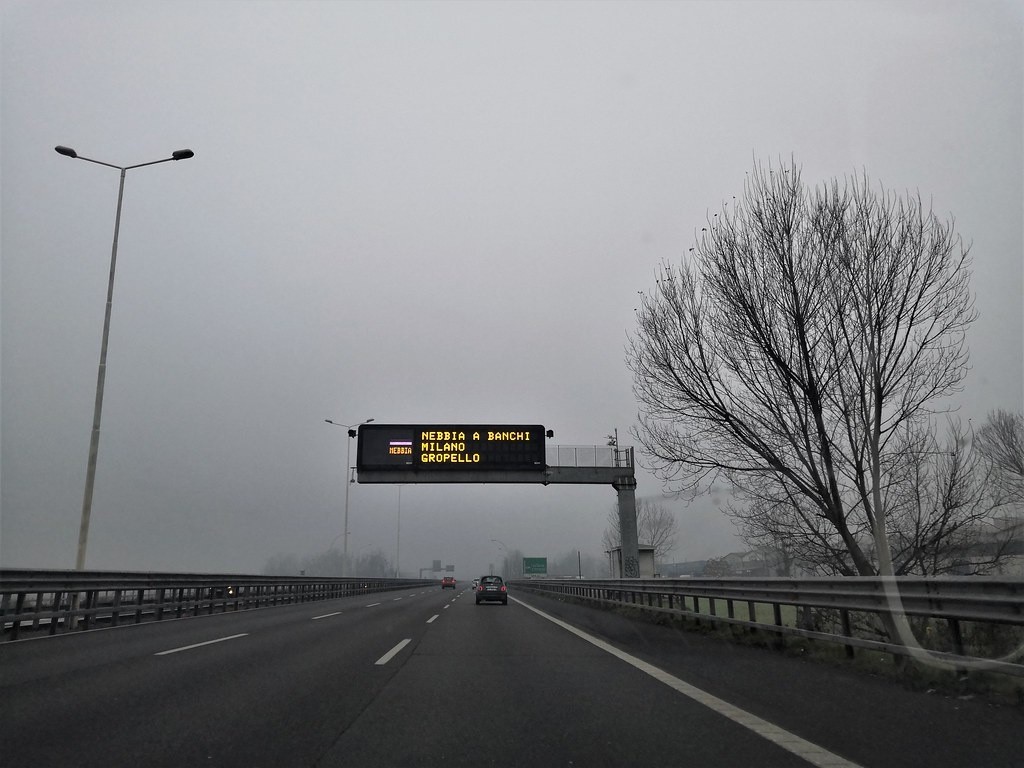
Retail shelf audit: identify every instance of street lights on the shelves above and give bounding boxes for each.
[325,418,374,576]
[55,145,195,628]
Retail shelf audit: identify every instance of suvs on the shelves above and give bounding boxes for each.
[476,576,507,605]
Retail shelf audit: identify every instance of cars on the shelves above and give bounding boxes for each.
[442,577,456,589]
[472,578,480,589]
